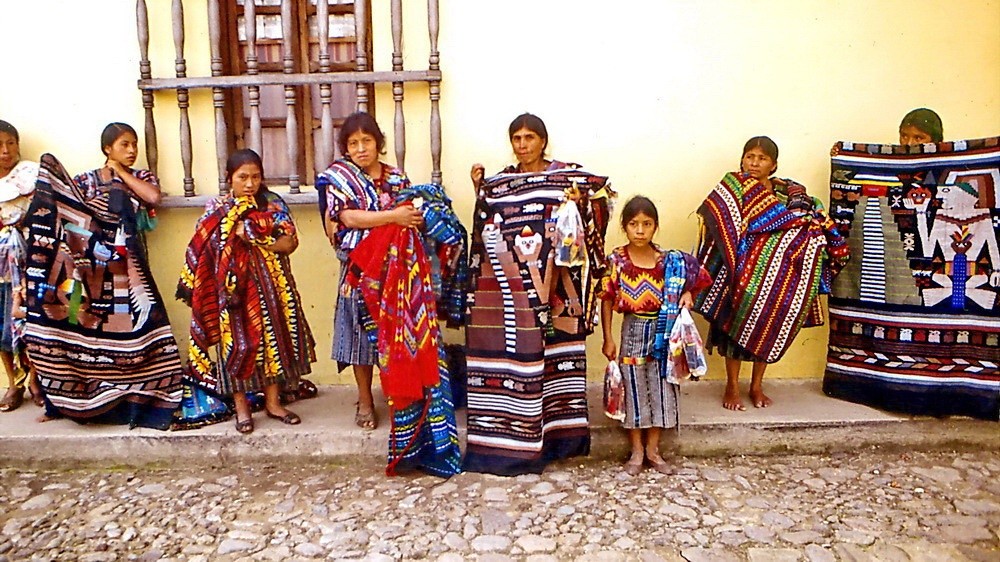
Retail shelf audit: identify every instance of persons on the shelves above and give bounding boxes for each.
[39,122,161,422]
[326,111,428,429]
[830,108,947,422]
[470,111,609,435]
[704,136,821,414]
[0,118,50,413]
[196,150,302,433]
[602,195,702,476]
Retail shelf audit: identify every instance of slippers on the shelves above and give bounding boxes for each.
[0,384,25,412]
[28,385,45,407]
[235,414,253,434]
[357,401,377,429]
[266,406,301,425]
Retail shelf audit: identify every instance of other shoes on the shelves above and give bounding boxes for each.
[645,454,673,474]
[625,452,646,476]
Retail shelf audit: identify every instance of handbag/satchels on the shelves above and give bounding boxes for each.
[0,226,28,291]
[666,305,708,384]
[603,360,626,423]
